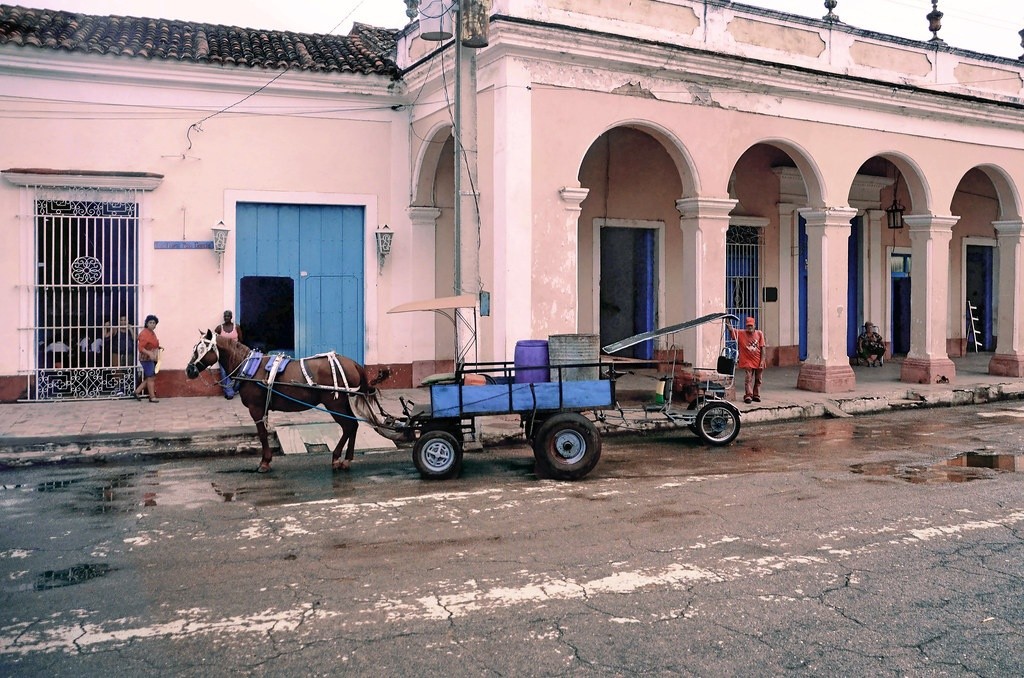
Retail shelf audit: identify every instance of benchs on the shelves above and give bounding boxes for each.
[691,348,737,413]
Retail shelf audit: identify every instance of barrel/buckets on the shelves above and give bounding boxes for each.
[514,339,548,383]
[548,333,600,382]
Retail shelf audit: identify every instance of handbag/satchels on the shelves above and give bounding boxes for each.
[155,349,161,374]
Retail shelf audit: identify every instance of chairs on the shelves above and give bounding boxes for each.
[857,325,885,366]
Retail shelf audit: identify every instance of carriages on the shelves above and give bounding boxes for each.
[185,325,616,482]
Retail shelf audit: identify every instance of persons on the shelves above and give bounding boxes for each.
[215,311,242,399]
[856,321,886,367]
[38,315,136,369]
[725,316,765,404]
[133,313,162,402]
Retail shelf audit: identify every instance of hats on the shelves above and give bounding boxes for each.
[746,317,755,326]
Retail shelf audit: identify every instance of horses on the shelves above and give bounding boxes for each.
[186,329,374,473]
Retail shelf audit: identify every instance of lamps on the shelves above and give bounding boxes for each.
[886,170,906,229]
[376,225,394,275]
[211,221,231,273]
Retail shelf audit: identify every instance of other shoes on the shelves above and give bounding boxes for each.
[149,396,160,403]
[132,389,141,402]
[869,360,879,367]
[744,399,751,404]
[752,397,761,402]
[224,394,233,400]
[113,389,123,396]
